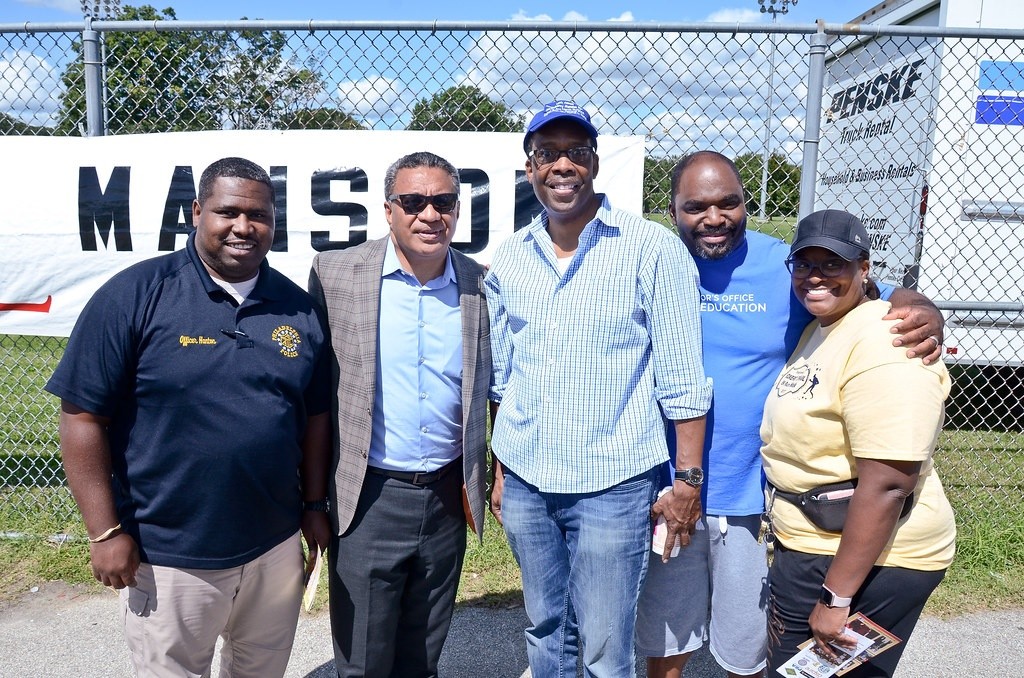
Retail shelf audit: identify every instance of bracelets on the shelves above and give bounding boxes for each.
[87,523,122,543]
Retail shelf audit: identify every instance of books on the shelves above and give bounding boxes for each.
[303,538,324,614]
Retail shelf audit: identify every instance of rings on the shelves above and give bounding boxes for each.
[931,335,942,348]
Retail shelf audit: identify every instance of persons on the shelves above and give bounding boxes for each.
[40,157,336,678]
[635,146,945,678]
[758,207,958,678]
[299,151,493,677]
[480,97,716,677]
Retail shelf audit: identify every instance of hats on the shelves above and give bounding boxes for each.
[786,209,871,263]
[523,99,598,159]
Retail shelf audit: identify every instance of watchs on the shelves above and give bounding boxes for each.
[673,465,708,488]
[818,581,853,612]
[300,495,331,517]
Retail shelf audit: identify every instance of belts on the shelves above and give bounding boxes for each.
[368,454,463,486]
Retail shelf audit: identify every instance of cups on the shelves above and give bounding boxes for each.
[652,486,681,557]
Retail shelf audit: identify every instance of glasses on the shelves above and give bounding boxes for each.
[389,193,459,215]
[785,257,846,279]
[528,146,596,165]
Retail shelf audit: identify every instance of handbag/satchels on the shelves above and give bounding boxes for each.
[765,478,914,535]
